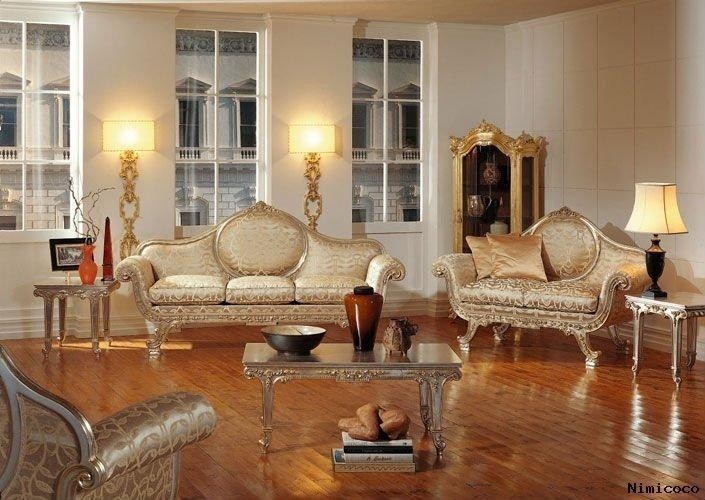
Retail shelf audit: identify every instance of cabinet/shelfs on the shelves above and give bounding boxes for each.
[447,117,548,254]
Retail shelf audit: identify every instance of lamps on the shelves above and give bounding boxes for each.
[287,121,340,233]
[627,184,690,299]
[100,117,159,262]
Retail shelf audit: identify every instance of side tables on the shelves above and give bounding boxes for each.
[620,290,704,393]
[30,276,121,363]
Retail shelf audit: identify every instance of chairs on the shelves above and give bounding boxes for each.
[0,344,219,500]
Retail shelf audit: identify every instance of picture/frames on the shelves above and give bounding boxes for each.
[47,237,96,271]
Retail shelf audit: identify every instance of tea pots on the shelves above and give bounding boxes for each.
[466,193,492,216]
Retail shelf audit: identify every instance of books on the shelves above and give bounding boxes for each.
[331,430,417,473]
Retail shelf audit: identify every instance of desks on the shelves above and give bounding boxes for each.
[243,341,466,462]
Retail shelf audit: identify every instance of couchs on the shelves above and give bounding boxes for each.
[430,205,651,371]
[111,196,408,368]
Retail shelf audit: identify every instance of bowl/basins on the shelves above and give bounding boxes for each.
[260,324,327,357]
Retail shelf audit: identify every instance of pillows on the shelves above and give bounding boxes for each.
[462,225,553,284]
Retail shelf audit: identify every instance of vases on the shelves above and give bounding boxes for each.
[342,285,383,353]
